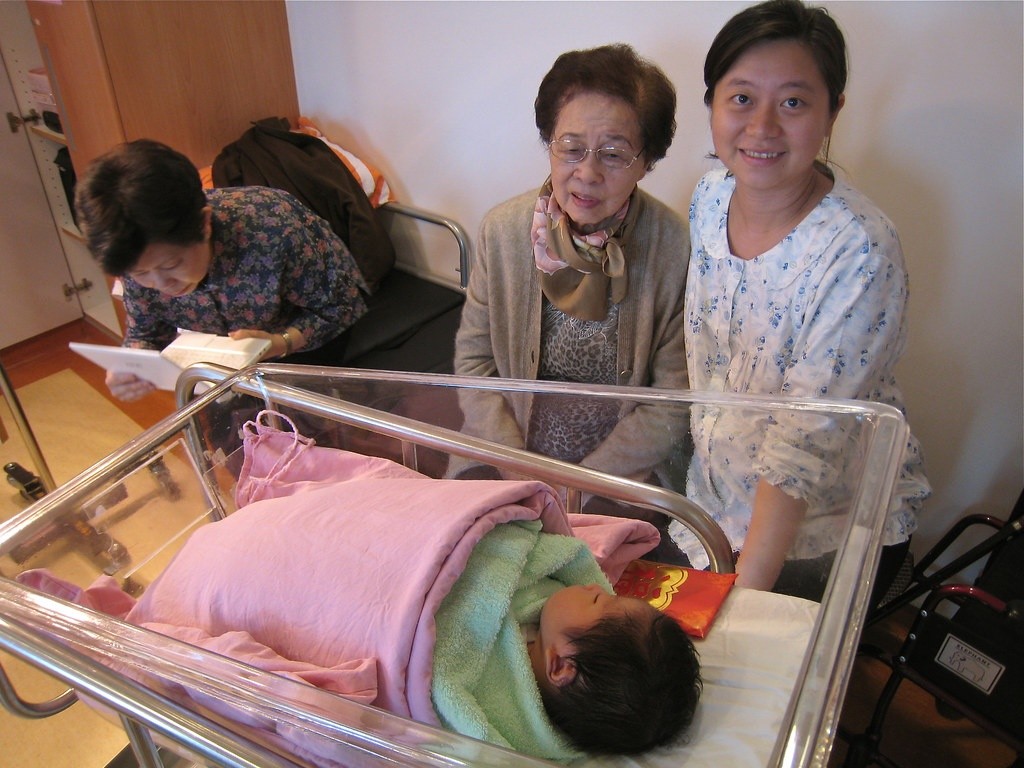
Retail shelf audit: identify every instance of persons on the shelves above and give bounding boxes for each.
[440,44,697,566]
[74,134,374,496]
[184,487,701,756]
[638,2,931,627]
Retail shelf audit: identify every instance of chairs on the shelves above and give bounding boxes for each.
[198,115,468,450]
[838,486,1024,768]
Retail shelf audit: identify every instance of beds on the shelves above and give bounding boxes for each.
[0,362,911,768]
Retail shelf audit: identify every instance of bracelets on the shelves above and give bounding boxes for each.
[276,330,292,359]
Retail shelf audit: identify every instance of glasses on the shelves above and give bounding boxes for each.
[548,139,644,169]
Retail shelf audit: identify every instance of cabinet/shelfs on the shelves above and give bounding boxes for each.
[0,0,301,345]
